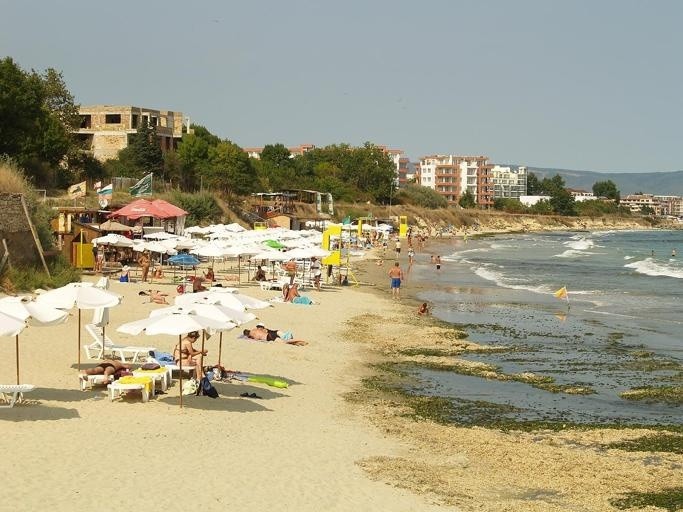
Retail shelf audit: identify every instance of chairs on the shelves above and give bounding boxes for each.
[0,384,33,408]
[79,324,196,403]
[260,276,291,292]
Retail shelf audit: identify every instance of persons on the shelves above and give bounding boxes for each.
[243,328,307,346]
[83,357,130,384]
[256,323,305,345]
[417,302,428,315]
[443,222,480,243]
[393,223,442,273]
[148,276,209,305]
[387,261,404,300]
[282,257,323,305]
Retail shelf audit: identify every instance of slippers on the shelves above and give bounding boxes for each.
[240,392,251,397]
[250,393,262,399]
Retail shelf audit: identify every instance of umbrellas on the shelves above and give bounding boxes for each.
[27,281,118,375]
[115,287,263,409]
[92,277,113,360]
[151,199,189,227]
[0,295,67,387]
[106,199,168,238]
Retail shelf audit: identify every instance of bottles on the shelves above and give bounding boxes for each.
[124,368,129,376]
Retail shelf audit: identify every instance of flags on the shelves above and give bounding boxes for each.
[342,216,350,224]
[552,286,566,299]
[68,181,85,199]
[125,173,151,197]
[93,181,101,190]
[95,184,112,200]
[367,212,372,221]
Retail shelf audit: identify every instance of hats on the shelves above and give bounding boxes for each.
[121,264,131,272]
